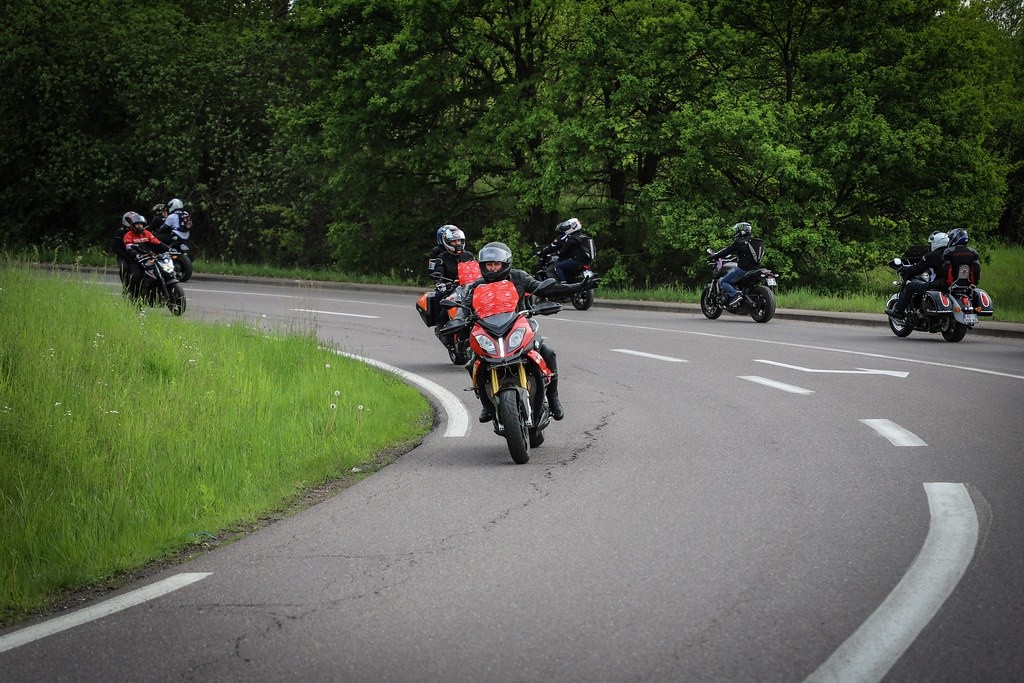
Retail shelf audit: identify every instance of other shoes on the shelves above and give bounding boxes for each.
[885,308,904,320]
[441,324,450,345]
[729,293,742,307]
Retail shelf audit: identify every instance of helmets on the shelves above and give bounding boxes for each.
[733,222,752,237]
[555,222,564,233]
[152,204,167,216]
[442,228,467,254]
[436,225,458,245]
[130,214,147,234]
[478,242,513,282]
[168,198,184,212]
[947,228,968,245]
[122,211,136,227]
[927,230,949,251]
[562,218,582,236]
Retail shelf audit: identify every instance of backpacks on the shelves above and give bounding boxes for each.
[173,211,193,232]
[573,233,596,260]
[740,236,764,265]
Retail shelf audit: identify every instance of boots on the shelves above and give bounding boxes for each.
[475,377,492,423]
[546,377,565,420]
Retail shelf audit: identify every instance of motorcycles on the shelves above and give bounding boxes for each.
[114,227,193,283]
[119,243,187,316]
[701,248,777,323]
[416,258,565,464]
[528,241,594,310]
[885,245,993,342]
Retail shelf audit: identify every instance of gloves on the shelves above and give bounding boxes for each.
[581,272,602,292]
[708,255,717,263]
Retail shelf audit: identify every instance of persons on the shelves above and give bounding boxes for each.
[706,222,763,305]
[911,228,981,309]
[441,241,602,420]
[428,226,475,334]
[115,211,178,304]
[154,198,189,243]
[145,204,168,235]
[885,230,950,322]
[535,217,593,283]
